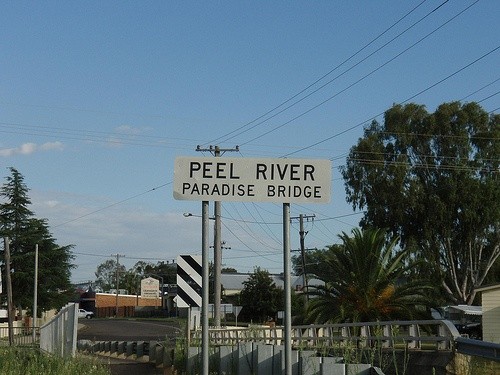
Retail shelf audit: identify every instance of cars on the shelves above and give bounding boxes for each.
[77,309,95,319]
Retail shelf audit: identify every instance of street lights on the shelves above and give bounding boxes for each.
[182,144,223,345]
